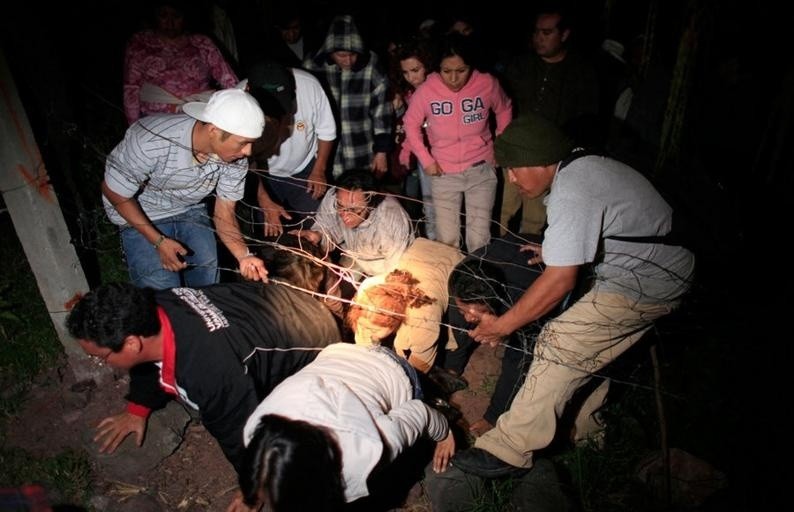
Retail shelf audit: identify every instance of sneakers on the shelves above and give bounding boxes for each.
[450,449,518,479]
[428,366,468,392]
[430,398,462,424]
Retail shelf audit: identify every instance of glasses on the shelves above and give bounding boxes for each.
[87,347,112,369]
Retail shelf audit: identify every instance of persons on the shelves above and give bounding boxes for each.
[64,1,696,512]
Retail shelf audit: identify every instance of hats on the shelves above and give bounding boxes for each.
[249,58,296,116]
[493,115,572,167]
[182,88,266,139]
